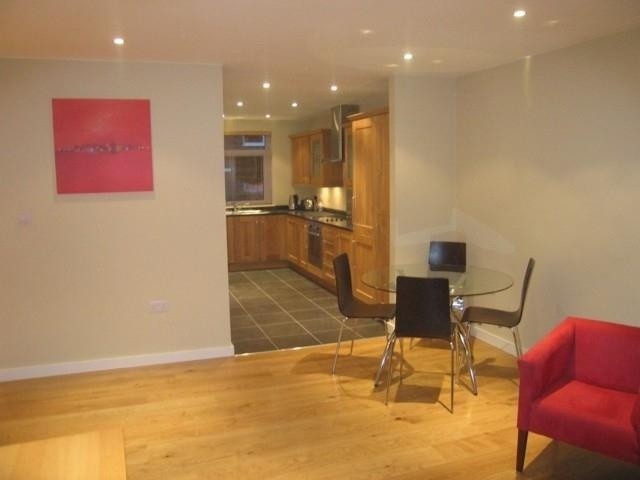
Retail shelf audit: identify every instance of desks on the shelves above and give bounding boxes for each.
[360,265,514,396]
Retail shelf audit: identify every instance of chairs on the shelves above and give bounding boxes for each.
[409,240,468,348]
[460,255,535,361]
[383,275,459,414]
[332,254,404,385]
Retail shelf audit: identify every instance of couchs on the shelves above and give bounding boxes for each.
[517,316,640,473]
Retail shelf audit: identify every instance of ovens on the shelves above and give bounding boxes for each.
[306,223,323,268]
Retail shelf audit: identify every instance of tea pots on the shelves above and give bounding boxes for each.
[288,193,298,210]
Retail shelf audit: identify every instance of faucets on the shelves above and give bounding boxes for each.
[233,201,249,211]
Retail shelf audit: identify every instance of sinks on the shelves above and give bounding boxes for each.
[242,210,270,215]
[226,212,242,215]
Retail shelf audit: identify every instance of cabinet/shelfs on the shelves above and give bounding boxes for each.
[311,130,344,188]
[290,133,311,185]
[287,216,352,296]
[227,212,291,271]
[345,108,389,305]
